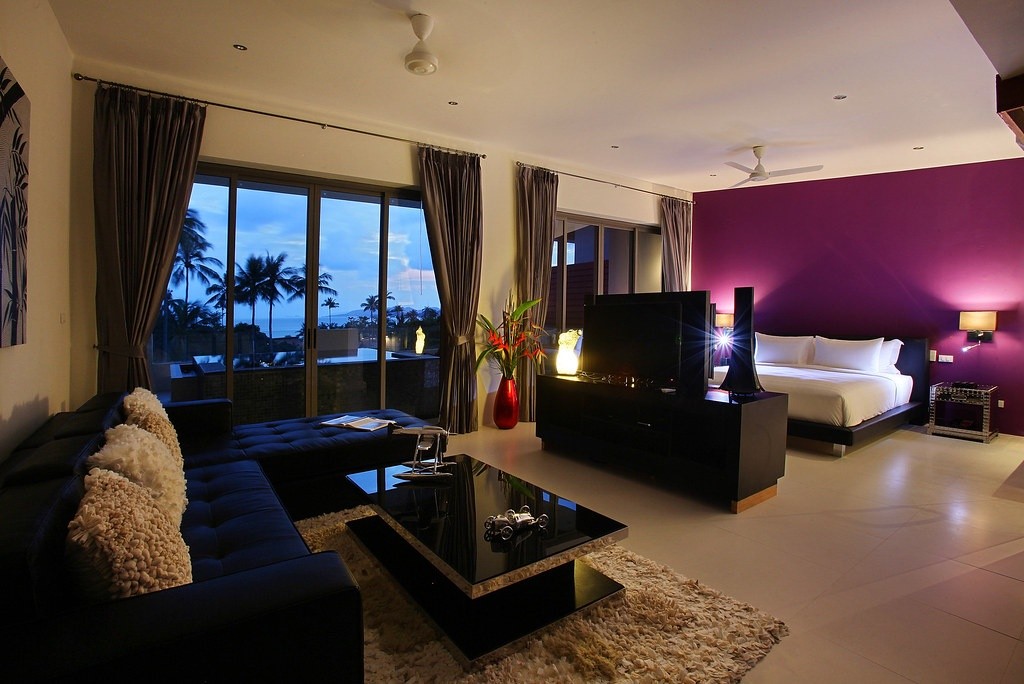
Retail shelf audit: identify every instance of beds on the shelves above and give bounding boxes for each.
[708,333,929,458]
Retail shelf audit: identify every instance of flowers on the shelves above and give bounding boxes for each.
[474,298,549,379]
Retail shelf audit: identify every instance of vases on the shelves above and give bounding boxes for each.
[493,377,519,429]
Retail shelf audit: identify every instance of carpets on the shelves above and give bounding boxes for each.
[293,503,790,684]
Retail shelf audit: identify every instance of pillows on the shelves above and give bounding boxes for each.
[754,331,904,375]
[66,388,193,600]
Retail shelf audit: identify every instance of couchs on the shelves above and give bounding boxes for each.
[77,390,447,521]
[0,410,445,684]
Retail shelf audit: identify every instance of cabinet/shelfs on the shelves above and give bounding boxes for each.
[536,373,789,514]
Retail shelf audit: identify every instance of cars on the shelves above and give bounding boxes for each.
[484,506,549,540]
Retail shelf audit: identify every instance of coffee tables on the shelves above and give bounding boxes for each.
[346,454,629,674]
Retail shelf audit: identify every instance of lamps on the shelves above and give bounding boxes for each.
[715,314,734,344]
[959,311,996,351]
[556,328,582,376]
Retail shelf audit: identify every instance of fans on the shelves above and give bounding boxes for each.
[722,146,823,187]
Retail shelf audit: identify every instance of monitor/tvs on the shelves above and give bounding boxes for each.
[581,301,683,380]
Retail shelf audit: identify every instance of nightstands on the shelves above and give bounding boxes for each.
[927,382,999,444]
